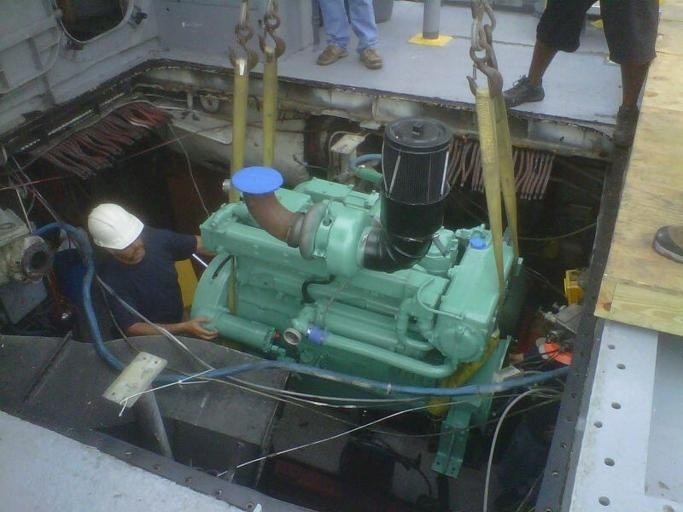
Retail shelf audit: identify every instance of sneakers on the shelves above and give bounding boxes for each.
[652,226,683,264]
[614,105,639,148]
[503,75,544,107]
[317,44,348,65]
[360,45,383,68]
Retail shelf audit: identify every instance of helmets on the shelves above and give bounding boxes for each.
[87,203,144,249]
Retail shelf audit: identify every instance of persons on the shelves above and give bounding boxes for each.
[480,336,571,506]
[88,203,225,340]
[316,1,391,71]
[654,224,682,262]
[496,1,660,146]
